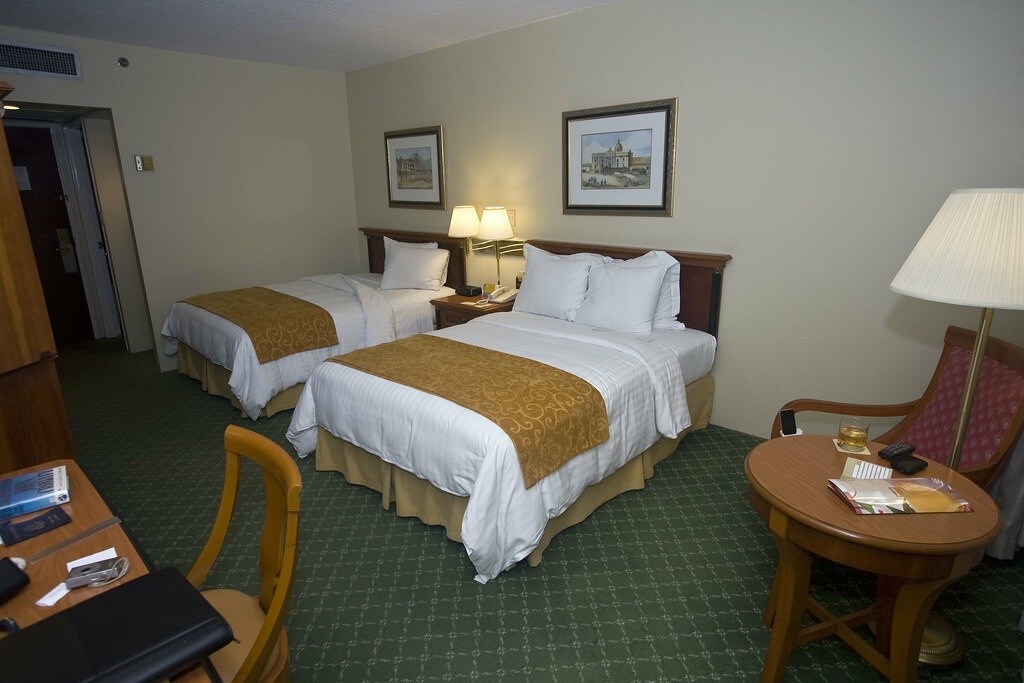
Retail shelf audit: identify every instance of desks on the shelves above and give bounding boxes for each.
[0,453,224,683]
[742,435,1003,682]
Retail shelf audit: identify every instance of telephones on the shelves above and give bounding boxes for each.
[488,285,520,304]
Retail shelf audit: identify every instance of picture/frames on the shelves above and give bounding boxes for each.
[384,124,446,211]
[560,97,680,217]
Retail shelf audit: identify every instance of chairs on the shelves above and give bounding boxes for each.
[191,426,307,683]
[769,326,1024,667]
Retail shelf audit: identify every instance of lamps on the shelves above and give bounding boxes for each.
[890,185,1024,665]
[478,206,525,302]
[448,204,495,253]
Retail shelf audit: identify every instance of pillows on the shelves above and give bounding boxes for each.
[383,237,441,256]
[512,243,686,335]
[380,247,451,291]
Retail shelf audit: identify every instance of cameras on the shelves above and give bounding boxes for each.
[66,556,122,590]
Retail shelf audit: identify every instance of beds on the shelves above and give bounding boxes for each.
[286,237,736,586]
[160,228,468,422]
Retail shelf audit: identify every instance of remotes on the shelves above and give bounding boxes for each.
[878,442,916,460]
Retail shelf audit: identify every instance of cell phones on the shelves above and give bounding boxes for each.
[779,408,796,436]
[890,455,928,475]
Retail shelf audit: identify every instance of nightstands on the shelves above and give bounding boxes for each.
[428,288,514,330]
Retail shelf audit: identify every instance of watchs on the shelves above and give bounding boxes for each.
[0,617,19,635]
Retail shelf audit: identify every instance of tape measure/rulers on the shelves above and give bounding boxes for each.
[30,516,122,563]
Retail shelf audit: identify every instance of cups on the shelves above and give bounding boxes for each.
[838,416,869,452]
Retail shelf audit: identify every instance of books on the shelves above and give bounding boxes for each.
[826,478,975,514]
[0,465,70,521]
